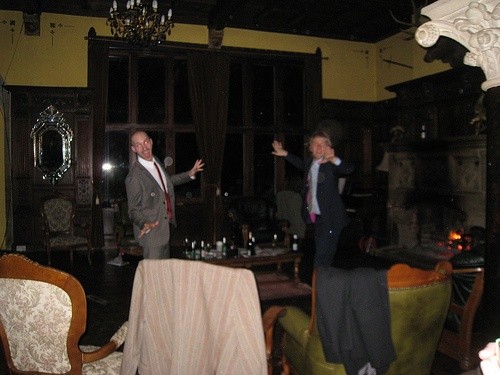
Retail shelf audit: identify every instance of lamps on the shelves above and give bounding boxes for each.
[106,0,175,49]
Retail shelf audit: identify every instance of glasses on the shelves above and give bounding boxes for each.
[134,141,150,148]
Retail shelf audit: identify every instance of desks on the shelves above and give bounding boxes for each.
[184,245,304,287]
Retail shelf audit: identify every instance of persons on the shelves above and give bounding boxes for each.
[270,130,353,288]
[125,129,205,259]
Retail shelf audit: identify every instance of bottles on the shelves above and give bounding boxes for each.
[183,231,298,260]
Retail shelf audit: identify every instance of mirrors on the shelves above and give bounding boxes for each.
[30,104,72,189]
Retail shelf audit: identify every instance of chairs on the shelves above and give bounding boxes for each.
[277,255,485,375]
[0,253,129,375]
[40,193,93,266]
[225,197,292,251]
[119,259,287,375]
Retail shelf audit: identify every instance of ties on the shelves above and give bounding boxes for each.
[308,169,314,212]
[153,161,173,221]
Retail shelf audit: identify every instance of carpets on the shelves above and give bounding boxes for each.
[254,273,314,299]
